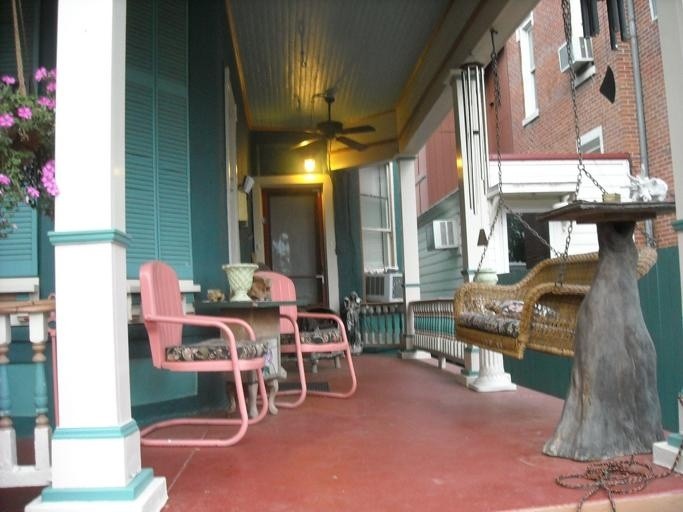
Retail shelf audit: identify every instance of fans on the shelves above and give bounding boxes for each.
[289,96,376,153]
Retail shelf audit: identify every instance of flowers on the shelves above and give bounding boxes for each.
[0,65,62,239]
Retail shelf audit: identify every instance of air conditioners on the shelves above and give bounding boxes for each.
[558,36,595,73]
[424,218,458,250]
[365,268,405,304]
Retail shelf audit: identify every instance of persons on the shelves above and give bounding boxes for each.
[344,292,364,349]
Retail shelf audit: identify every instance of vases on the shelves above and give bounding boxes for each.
[220,262,259,303]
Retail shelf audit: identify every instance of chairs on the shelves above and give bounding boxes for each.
[133,261,271,447]
[253,271,358,409]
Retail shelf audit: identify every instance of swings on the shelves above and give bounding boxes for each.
[453,1,658,360]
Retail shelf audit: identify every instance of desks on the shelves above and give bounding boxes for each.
[191,300,297,418]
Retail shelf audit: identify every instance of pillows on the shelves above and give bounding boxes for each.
[481,296,561,324]
